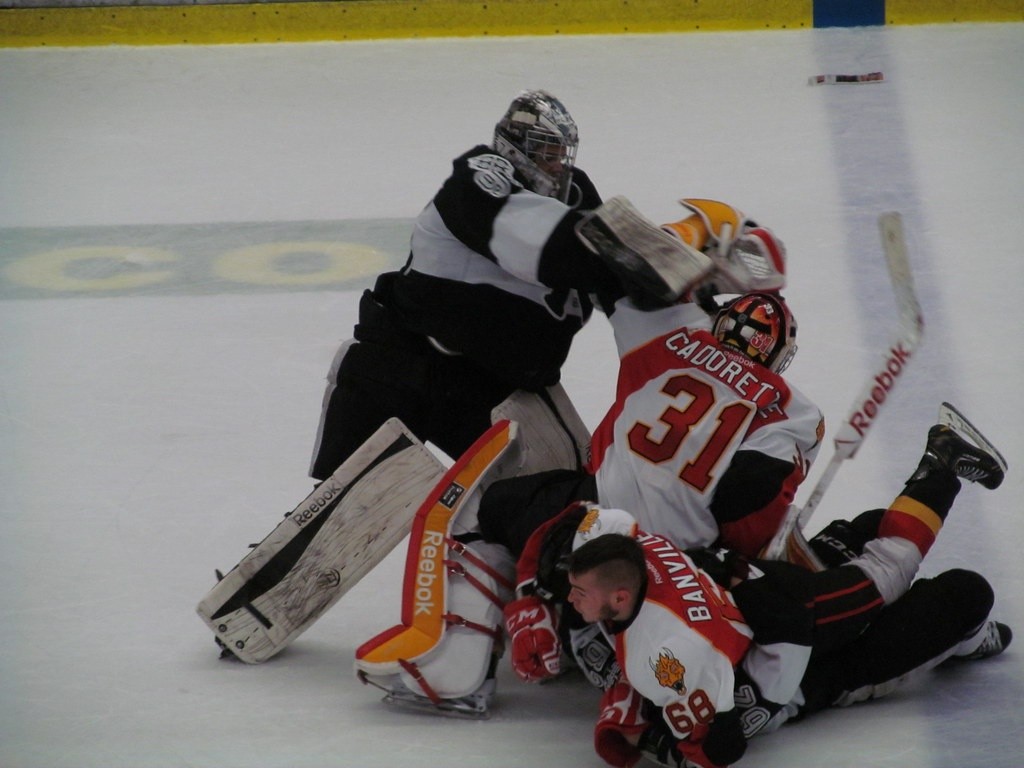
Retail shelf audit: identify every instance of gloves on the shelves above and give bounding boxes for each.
[595,670,649,768]
[504,597,561,684]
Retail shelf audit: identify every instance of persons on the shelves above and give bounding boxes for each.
[197,87,1014,768]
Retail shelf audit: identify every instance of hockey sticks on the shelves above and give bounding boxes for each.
[771,208,932,560]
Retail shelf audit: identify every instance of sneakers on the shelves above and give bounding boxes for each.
[380,672,496,720]
[905,402,1008,490]
[947,621,1012,664]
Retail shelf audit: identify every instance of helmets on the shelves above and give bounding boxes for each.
[494,90,581,197]
[711,292,798,376]
